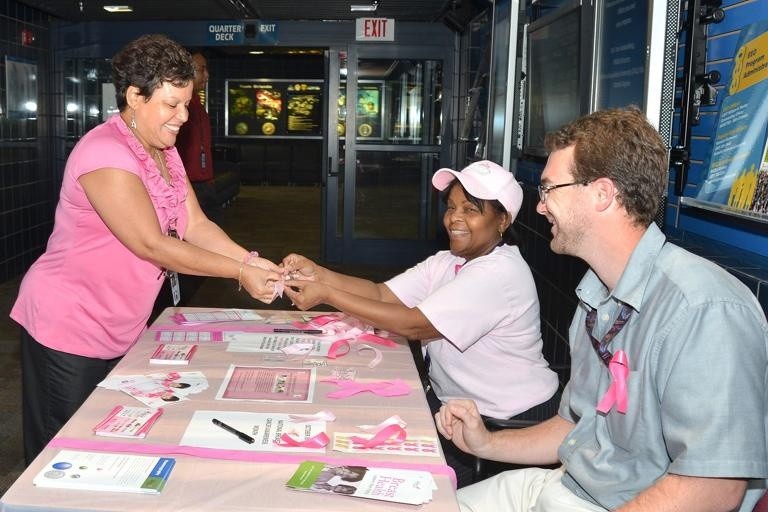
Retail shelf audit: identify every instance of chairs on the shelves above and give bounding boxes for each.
[474,366,571,474]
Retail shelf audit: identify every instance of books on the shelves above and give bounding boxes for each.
[33,450,175,491]
[92,404,161,435]
[32,461,177,495]
[94,407,164,439]
[148,343,198,365]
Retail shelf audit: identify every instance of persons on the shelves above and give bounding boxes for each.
[279,161,561,488]
[433,102,766,511]
[148,52,216,330]
[313,481,357,494]
[5,35,285,469]
[318,465,368,485]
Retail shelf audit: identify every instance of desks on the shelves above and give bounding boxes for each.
[0,306,461,512]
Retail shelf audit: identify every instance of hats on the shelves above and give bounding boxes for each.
[431,160,525,225]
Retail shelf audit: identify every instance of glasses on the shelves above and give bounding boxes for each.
[537,182,584,204]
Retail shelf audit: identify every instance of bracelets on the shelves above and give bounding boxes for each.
[237,263,243,292]
[244,251,259,264]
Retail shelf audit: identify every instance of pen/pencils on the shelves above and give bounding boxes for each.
[274,329,322,333]
[212,418,255,444]
